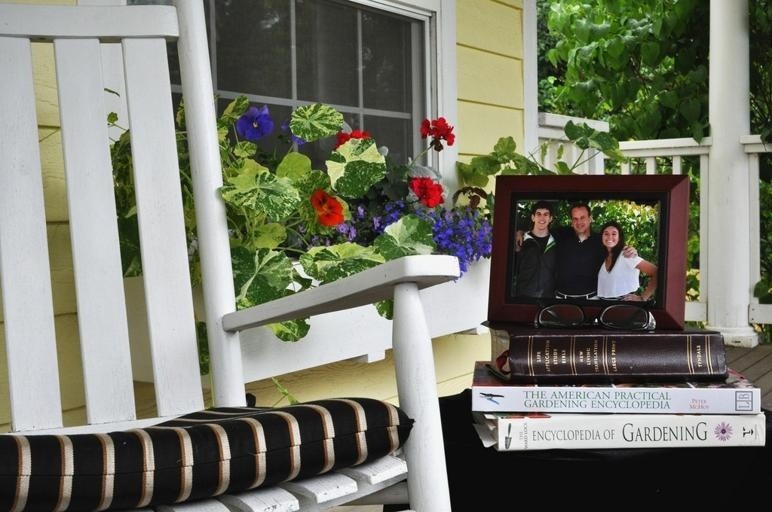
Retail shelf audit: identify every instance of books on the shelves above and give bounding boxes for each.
[470,359,763,415]
[474,414,768,448]
[480,316,727,381]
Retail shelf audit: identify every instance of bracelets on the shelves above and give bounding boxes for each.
[637,293,645,301]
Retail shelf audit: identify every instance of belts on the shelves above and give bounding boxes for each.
[555,292,597,299]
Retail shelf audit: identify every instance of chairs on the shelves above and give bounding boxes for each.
[0,0,463,512]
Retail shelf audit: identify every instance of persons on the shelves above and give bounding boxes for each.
[516,204,639,302]
[597,221,658,301]
[514,200,556,301]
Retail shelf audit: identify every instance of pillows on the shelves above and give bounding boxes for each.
[0,397,416,512]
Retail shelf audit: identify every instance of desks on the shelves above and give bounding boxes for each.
[381,387,772,512]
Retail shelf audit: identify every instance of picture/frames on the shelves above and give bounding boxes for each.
[487,174,690,334]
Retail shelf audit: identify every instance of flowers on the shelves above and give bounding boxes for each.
[107,84,632,378]
